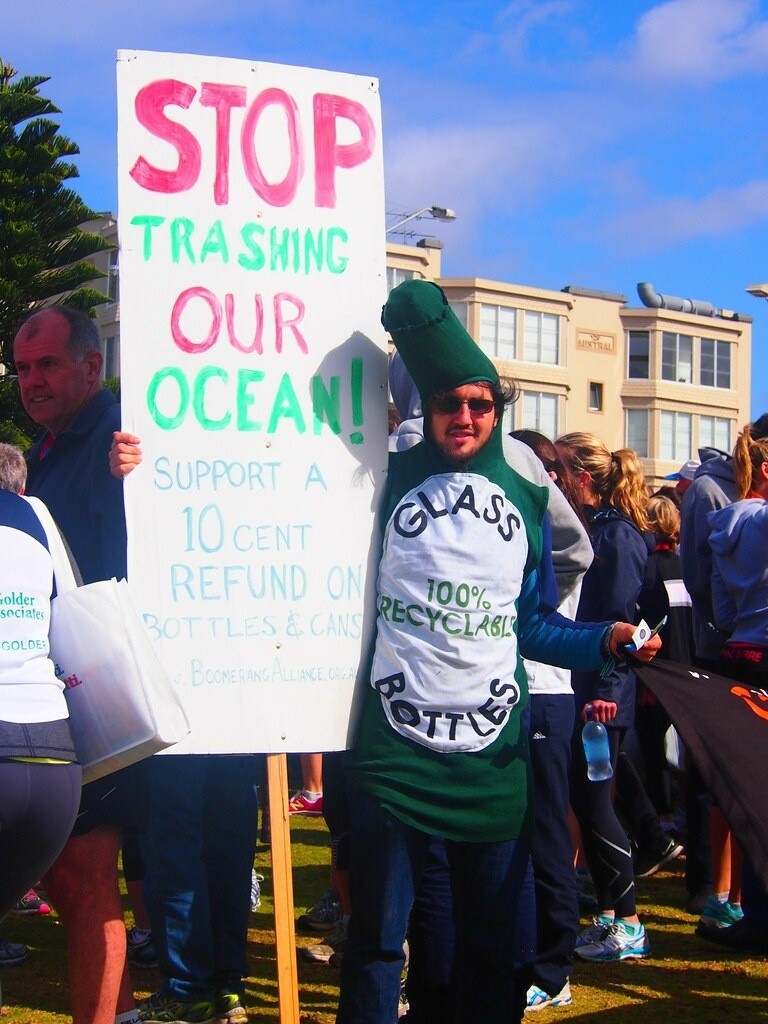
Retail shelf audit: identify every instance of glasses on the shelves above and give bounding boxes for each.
[434,393,497,414]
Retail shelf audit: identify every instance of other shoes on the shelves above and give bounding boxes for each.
[574,871,598,906]
[659,813,676,833]
[0,937,29,965]
[694,917,768,950]
[249,874,264,912]
[397,978,410,1020]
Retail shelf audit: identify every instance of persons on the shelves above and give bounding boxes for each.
[109,280,662,1024]
[0,487,82,927]
[0,442,29,495]
[127,402,768,1024]
[13,306,141,1024]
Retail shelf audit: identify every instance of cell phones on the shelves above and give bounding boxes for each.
[648,615,667,642]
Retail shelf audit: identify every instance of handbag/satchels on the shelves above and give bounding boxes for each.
[20,494,191,787]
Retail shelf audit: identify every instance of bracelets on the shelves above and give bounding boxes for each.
[605,621,622,667]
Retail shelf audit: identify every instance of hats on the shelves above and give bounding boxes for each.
[664,460,701,482]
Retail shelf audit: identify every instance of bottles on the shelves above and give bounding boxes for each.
[581,705,614,781]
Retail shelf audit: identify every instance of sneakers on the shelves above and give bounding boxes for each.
[214,993,250,1024]
[633,837,684,877]
[716,902,744,929]
[10,889,51,914]
[574,923,652,963]
[699,895,723,927]
[297,888,346,967]
[136,991,217,1024]
[575,914,615,949]
[127,924,159,969]
[524,975,572,1012]
[32,880,43,889]
[288,790,324,815]
